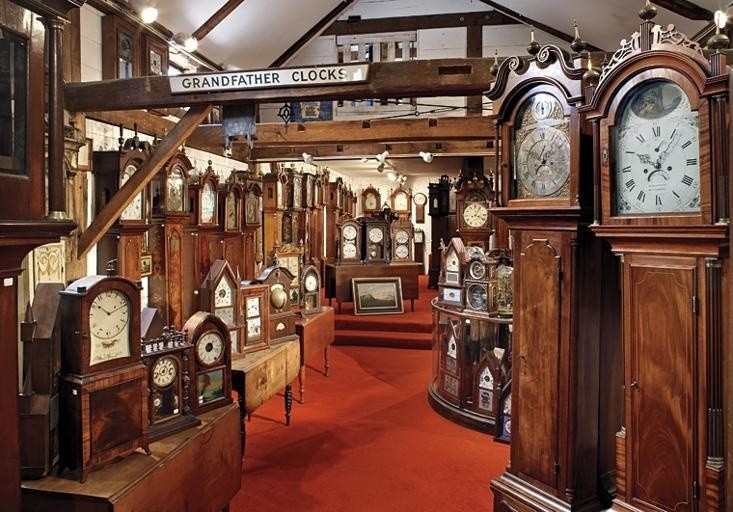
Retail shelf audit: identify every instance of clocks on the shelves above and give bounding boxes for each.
[138,307,234,443]
[93,122,269,361]
[452,170,497,252]
[479,13,620,511]
[1,0,80,512]
[432,236,519,446]
[266,175,452,346]
[101,14,171,117]
[578,0,732,510]
[58,259,154,484]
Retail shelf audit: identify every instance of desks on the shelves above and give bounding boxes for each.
[20,402,244,511]
[295,306,337,404]
[229,333,302,466]
